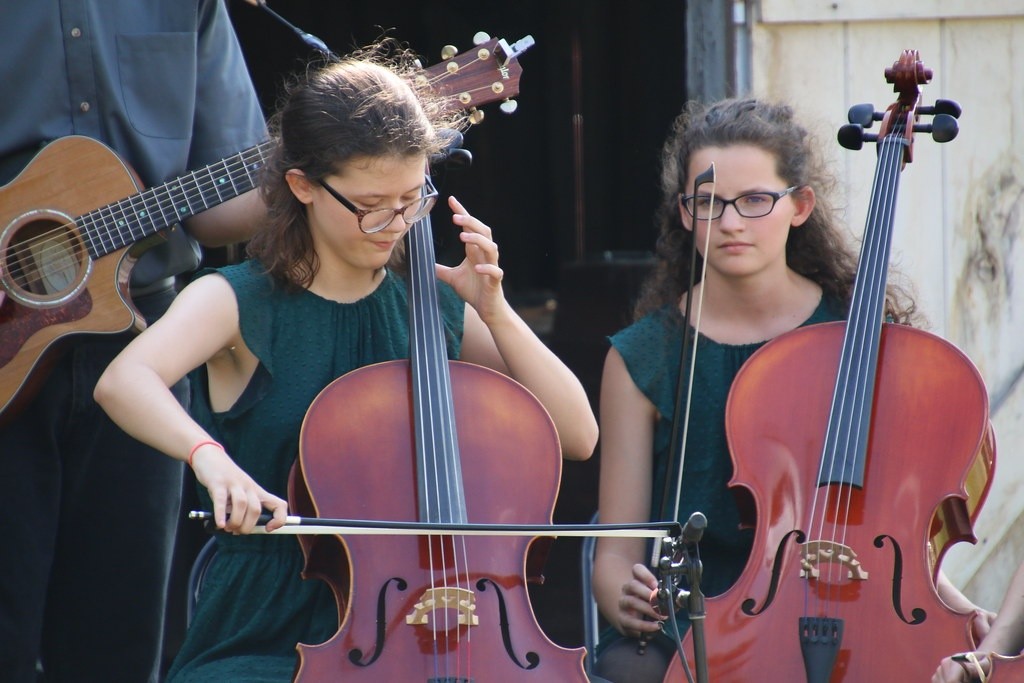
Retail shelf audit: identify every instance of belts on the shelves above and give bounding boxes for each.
[132,275,176,300]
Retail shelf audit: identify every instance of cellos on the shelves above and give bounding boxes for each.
[660,50,999,683]
[286,62,592,683]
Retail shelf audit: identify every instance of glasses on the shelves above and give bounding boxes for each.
[318,177,439,234]
[681,185,800,221]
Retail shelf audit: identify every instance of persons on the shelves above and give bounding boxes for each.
[932,559,1024,683]
[93,59,598,683]
[590,96,998,683]
[0,0,278,683]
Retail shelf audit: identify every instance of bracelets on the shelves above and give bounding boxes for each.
[189,440,224,466]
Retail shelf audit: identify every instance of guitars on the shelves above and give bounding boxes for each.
[1,28,537,408]
